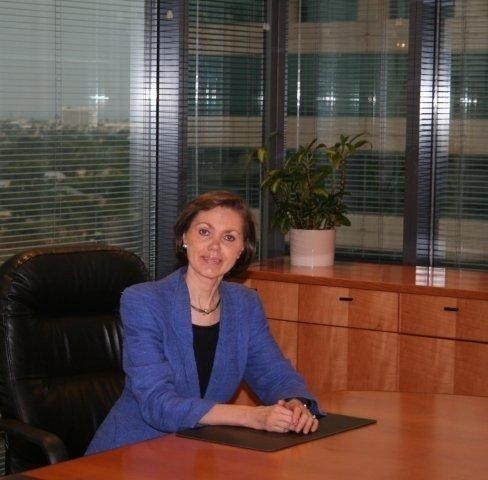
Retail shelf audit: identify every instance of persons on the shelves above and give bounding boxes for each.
[84,190,328,458]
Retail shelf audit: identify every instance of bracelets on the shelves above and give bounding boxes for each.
[299,398,320,421]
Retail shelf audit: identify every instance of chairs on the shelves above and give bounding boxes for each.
[1,242,155,476]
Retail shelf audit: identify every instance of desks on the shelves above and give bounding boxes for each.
[0,389,487,479]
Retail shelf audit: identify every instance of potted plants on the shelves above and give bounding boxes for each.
[257,132,373,267]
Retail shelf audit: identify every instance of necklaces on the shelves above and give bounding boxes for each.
[190,297,221,315]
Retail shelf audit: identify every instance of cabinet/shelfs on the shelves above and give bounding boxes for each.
[229,256,488,407]
